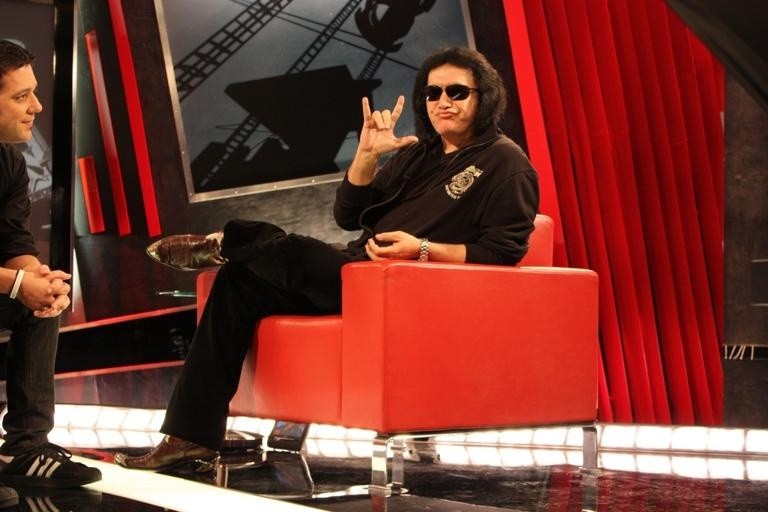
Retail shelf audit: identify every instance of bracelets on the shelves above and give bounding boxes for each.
[417,236,428,263]
[7,267,28,301]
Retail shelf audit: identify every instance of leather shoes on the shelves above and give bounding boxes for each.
[145,231,229,272]
[113,434,220,473]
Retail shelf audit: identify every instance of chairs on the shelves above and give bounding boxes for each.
[196,213,602,489]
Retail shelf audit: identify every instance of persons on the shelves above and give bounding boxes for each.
[0,35,101,509]
[109,43,538,474]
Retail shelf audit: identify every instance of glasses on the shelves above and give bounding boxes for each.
[422,84,478,101]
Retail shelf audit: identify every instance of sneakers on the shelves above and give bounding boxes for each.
[0,483,19,508]
[0,442,102,489]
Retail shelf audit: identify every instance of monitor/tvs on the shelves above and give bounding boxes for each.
[154,0,478,205]
[267,419,309,452]
[0,0,78,313]
[266,450,314,490]
